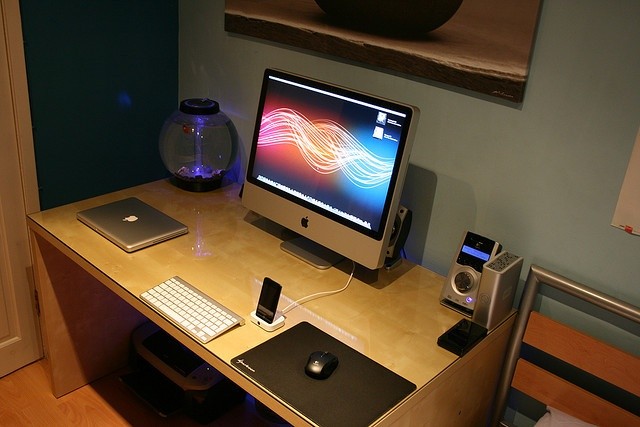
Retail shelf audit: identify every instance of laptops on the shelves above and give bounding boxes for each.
[77,197,188,252]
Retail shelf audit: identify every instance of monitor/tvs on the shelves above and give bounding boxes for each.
[242,67,420,270]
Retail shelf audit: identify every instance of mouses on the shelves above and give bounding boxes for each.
[305,351,339,379]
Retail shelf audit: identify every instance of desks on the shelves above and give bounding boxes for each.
[26,180,517,427]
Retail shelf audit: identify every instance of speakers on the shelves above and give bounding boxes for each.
[438,229,502,318]
[386,205,412,269]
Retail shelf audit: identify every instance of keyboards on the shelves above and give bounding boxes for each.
[140,276,245,343]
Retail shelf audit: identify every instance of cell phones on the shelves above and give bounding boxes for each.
[256,277,282,324]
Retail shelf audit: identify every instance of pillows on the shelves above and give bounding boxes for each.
[535,405,595,426]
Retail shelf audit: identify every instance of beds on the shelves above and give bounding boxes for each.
[490,264,639,426]
